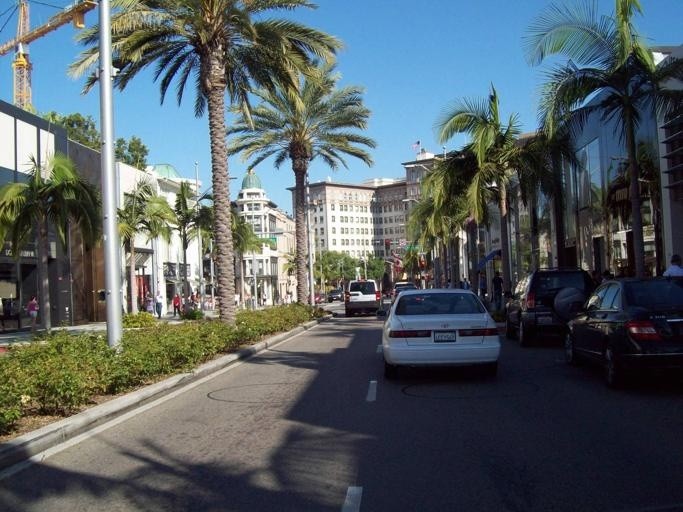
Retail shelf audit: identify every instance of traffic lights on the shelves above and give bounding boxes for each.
[385,239,391,251]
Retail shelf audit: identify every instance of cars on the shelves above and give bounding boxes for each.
[375,289,501,371]
[560,277,683,389]
[308,279,418,316]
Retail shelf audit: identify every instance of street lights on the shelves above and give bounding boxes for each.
[403,164,433,175]
[401,198,419,205]
[189,176,238,210]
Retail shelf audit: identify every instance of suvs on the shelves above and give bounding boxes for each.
[503,265,596,348]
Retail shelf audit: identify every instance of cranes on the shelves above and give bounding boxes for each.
[0,1,98,115]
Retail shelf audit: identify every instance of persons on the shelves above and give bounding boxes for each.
[145,291,201,319]
[446,254,683,313]
[27,296,40,332]
